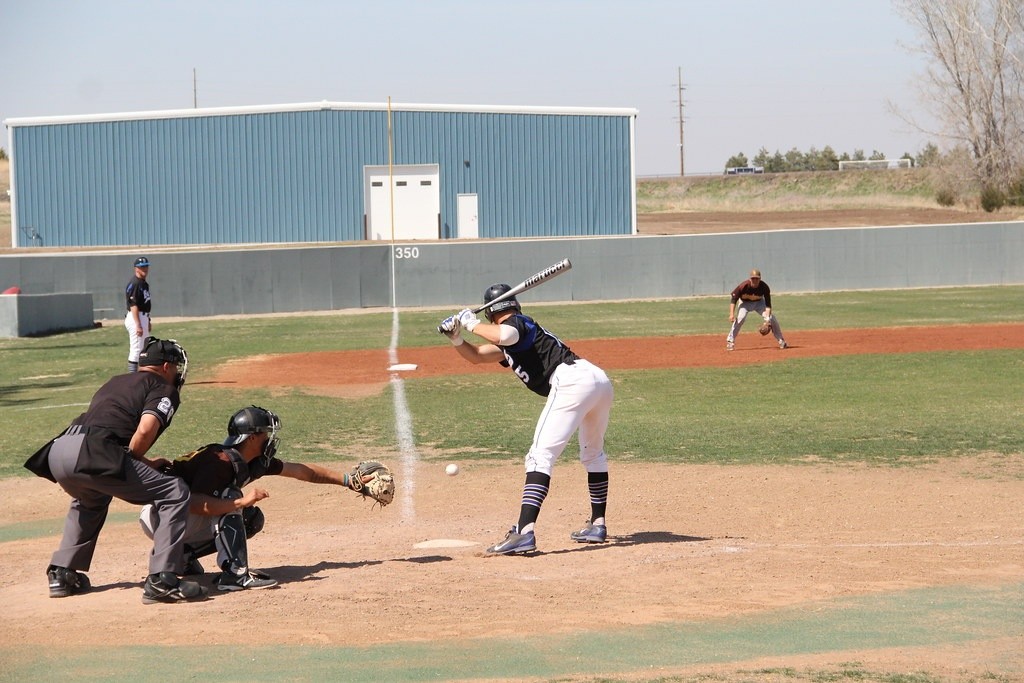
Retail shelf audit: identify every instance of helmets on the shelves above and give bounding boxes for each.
[485,283,522,324]
[750,270,761,278]
[134,257,149,267]
[221,407,276,446]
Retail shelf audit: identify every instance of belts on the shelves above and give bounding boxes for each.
[128,309,151,317]
[63,426,91,434]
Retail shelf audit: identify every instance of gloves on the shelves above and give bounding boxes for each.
[443,316,465,348]
[456,308,478,332]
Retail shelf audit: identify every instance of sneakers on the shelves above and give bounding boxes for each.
[217,570,277,592]
[485,525,536,554]
[48,567,89,597]
[571,523,607,542]
[181,560,204,576]
[141,575,210,603]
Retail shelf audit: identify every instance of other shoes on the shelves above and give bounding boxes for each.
[727,343,735,351]
[779,339,788,348]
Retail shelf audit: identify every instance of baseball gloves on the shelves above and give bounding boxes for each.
[758,321,773,336]
[350,458,395,505]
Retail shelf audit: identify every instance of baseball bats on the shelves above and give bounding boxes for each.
[437,257,572,336]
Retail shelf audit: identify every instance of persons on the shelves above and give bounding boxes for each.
[726,270,786,351]
[124,257,151,373]
[139,404,394,591]
[24,337,208,605]
[441,283,613,555]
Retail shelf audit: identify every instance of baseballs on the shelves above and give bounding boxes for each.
[446,464,459,476]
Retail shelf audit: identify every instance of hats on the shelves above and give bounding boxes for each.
[139,341,189,367]
